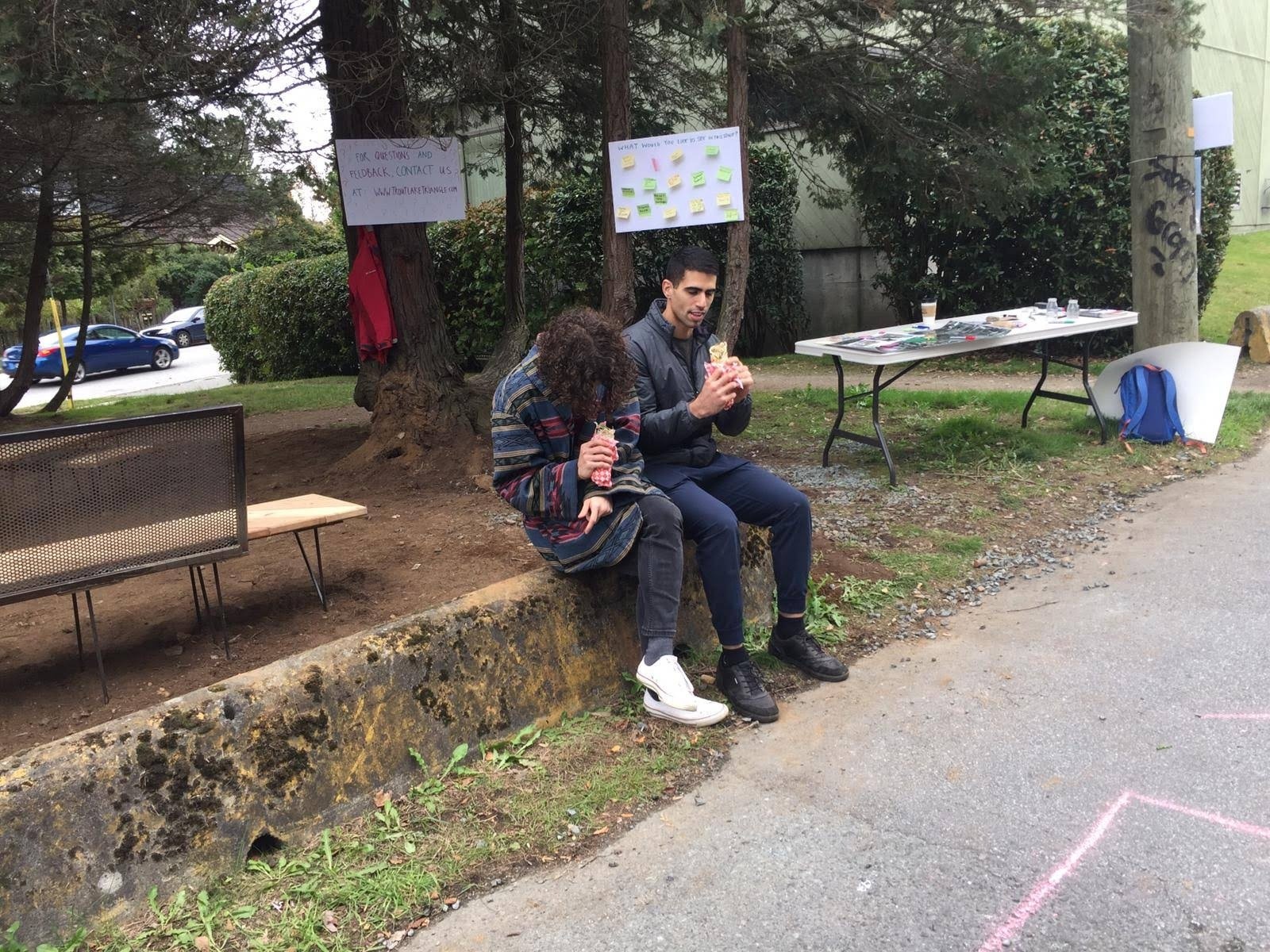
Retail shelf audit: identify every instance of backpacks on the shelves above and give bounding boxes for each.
[1113,362,1207,455]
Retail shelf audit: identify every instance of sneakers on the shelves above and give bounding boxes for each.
[766,624,850,682]
[716,653,779,722]
[643,685,728,728]
[635,653,699,712]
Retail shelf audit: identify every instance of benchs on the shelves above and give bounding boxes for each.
[0,404,370,703]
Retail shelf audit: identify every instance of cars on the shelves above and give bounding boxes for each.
[4,323,180,385]
[138,305,210,349]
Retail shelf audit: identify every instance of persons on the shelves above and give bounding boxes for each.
[619,246,851,722]
[490,306,730,727]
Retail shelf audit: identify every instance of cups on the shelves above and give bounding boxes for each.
[921,298,937,323]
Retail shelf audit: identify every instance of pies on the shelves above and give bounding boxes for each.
[594,421,615,439]
[709,342,728,365]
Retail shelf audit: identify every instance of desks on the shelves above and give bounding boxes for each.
[794,303,1139,487]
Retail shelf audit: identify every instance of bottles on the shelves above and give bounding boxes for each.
[1046,298,1058,319]
[1067,299,1080,318]
